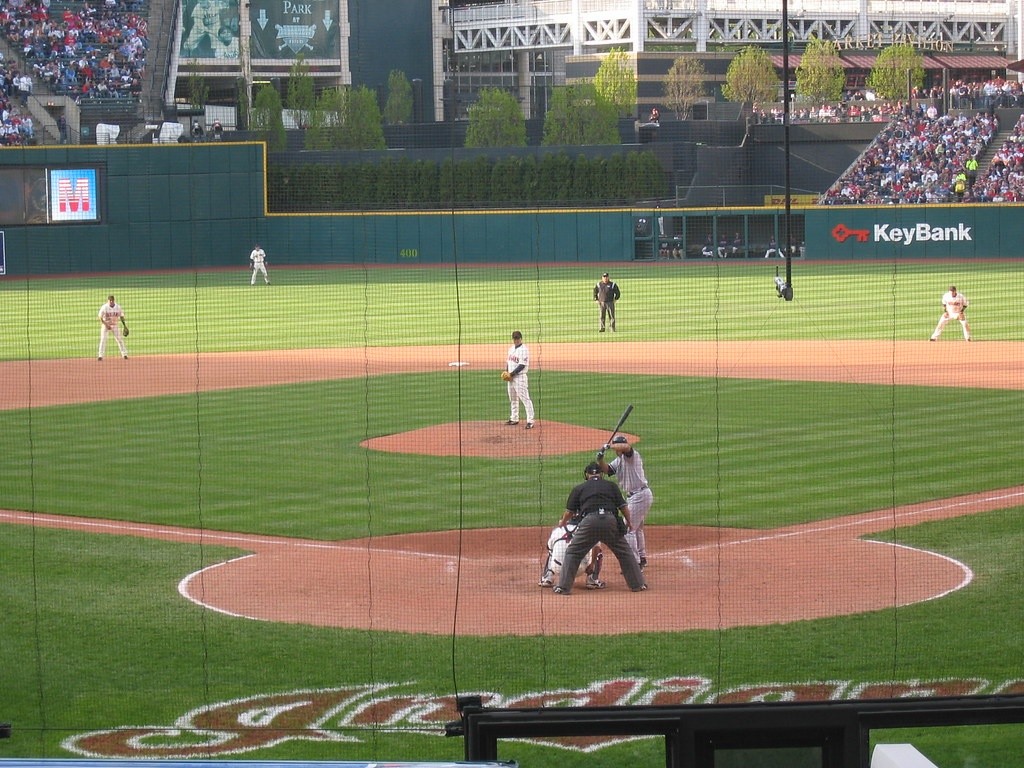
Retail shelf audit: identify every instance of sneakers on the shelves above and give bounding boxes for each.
[505,420,519,425]
[552,585,570,595]
[639,558,648,572]
[586,574,605,589]
[538,573,555,587]
[526,423,534,429]
[633,583,648,592]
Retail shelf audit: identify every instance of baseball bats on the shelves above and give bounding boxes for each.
[602,404,634,453]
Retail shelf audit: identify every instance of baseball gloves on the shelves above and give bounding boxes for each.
[959,314,965,320]
[500,371,513,381]
[123,327,129,337]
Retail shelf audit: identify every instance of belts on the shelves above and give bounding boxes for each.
[628,486,648,498]
[554,559,562,566]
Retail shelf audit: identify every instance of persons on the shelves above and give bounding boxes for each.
[702,232,742,258]
[752,76,1024,205]
[0,0,151,146]
[501,331,534,429]
[184,0,239,58]
[765,235,797,258]
[538,436,652,595]
[650,109,660,123]
[98,296,128,360]
[210,118,223,141]
[593,273,620,332]
[249,245,270,286]
[191,119,203,142]
[660,235,683,259]
[929,286,972,341]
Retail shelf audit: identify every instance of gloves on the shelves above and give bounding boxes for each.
[603,444,610,450]
[596,452,604,459]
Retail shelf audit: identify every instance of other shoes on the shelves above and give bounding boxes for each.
[967,338,972,342]
[599,328,605,332]
[930,339,937,341]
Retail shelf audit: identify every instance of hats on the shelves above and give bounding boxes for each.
[512,331,522,338]
[949,286,956,291]
[612,436,627,443]
[602,272,608,277]
[584,463,601,475]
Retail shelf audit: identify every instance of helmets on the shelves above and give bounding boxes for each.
[570,512,586,525]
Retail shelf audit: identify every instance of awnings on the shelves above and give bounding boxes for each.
[932,55,1009,108]
[767,55,856,68]
[1006,59,1024,74]
[845,55,946,114]
